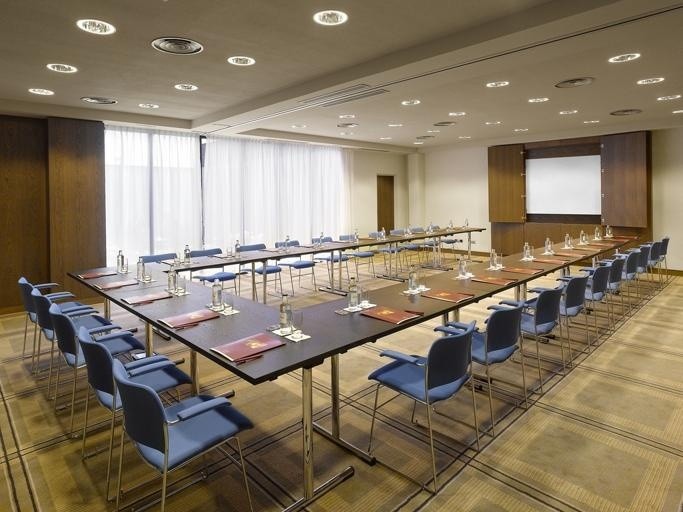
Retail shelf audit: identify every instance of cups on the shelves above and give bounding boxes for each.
[176,275,187,294]
[466,259,472,275]
[122,258,129,274]
[222,289,234,313]
[142,267,152,283]
[360,286,370,304]
[290,311,303,332]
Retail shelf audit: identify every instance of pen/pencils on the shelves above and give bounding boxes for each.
[173,323,199,328]
[404,310,425,316]
[235,354,265,362]
[130,301,154,305]
[102,287,122,290]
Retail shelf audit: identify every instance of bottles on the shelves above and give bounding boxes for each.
[409,264,418,290]
[348,276,360,308]
[279,293,292,334]
[211,278,224,311]
[117,250,124,273]
[136,256,145,281]
[172,217,611,268]
[459,252,466,277]
[167,263,177,294]
